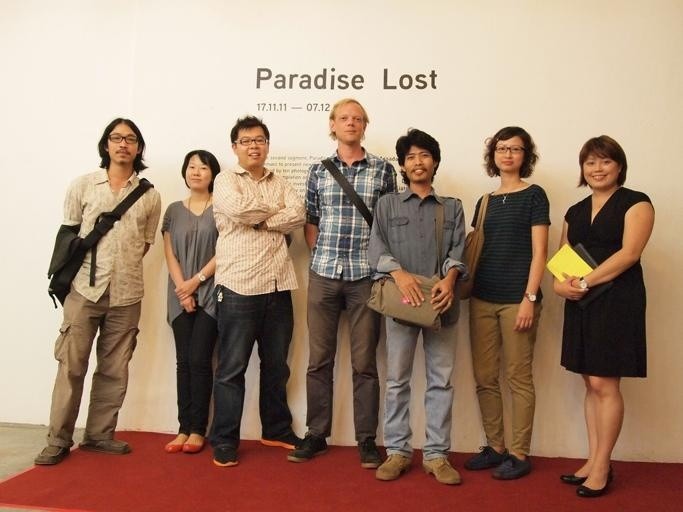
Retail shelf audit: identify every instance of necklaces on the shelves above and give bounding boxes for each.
[187,195,211,214]
[496,182,528,205]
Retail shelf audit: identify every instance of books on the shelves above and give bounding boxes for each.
[544,243,611,304]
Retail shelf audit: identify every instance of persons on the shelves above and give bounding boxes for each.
[161,151,218,456]
[462,126,546,481]
[210,117,306,466]
[368,130,466,483]
[35,119,158,468]
[286,99,394,470]
[553,134,653,499]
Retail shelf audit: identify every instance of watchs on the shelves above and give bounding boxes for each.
[524,291,539,305]
[197,270,207,284]
[578,279,589,294]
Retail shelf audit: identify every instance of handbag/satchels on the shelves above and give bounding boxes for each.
[367,195,444,330]
[457,194,489,301]
[47,178,154,303]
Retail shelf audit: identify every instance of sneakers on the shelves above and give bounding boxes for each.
[375,453,411,480]
[213,443,240,466]
[423,455,462,485]
[260,429,305,450]
[288,433,328,462]
[491,452,532,479]
[78,436,130,453]
[359,437,380,468]
[463,445,508,470]
[35,444,69,465]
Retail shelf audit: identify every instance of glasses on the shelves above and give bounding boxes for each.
[495,146,526,153]
[234,137,270,148]
[107,133,140,145]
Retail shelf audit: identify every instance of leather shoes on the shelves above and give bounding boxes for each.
[576,464,613,497]
[183,443,203,453]
[560,473,587,483]
[166,443,182,451]
